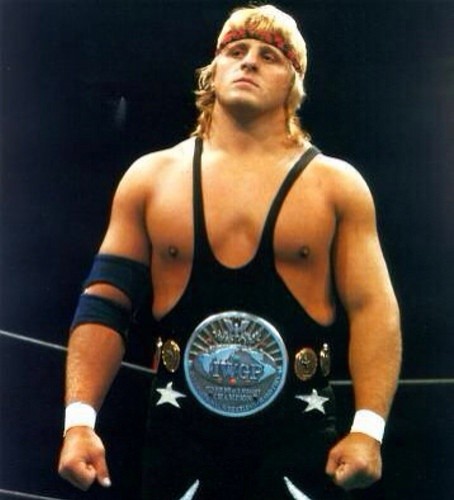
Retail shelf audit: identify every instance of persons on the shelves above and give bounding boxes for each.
[57,3,405,499]
